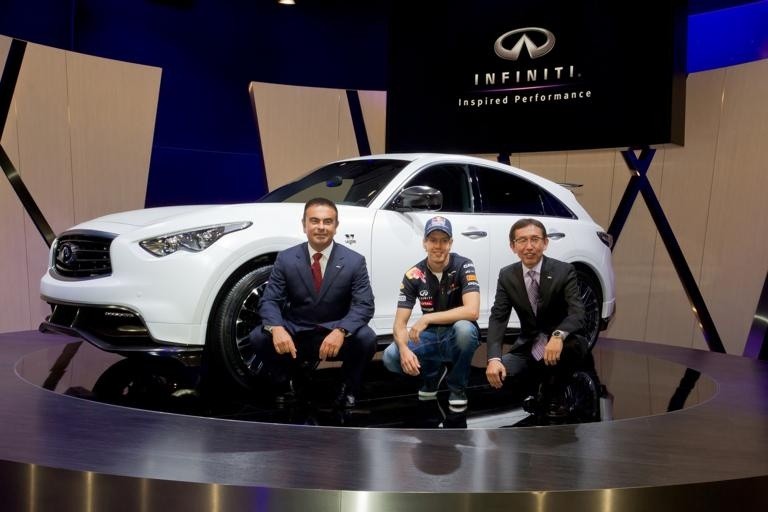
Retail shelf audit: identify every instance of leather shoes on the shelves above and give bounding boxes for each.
[548,398,564,419]
[276,390,316,405]
[521,393,538,412]
[335,382,356,409]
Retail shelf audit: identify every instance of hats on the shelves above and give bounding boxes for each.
[424,216,453,238]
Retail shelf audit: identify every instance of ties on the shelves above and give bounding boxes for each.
[527,269,549,363]
[311,252,323,293]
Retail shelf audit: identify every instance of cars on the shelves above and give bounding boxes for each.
[37,152,616,397]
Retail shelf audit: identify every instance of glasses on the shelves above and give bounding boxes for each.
[512,235,545,243]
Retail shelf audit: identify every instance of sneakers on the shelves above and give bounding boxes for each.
[418,364,448,398]
[449,389,469,407]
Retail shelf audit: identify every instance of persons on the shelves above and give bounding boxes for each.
[486,219,588,419]
[382,215,480,406]
[247,197,379,408]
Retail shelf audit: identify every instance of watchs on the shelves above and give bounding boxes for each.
[334,325,349,334]
[552,330,566,341]
